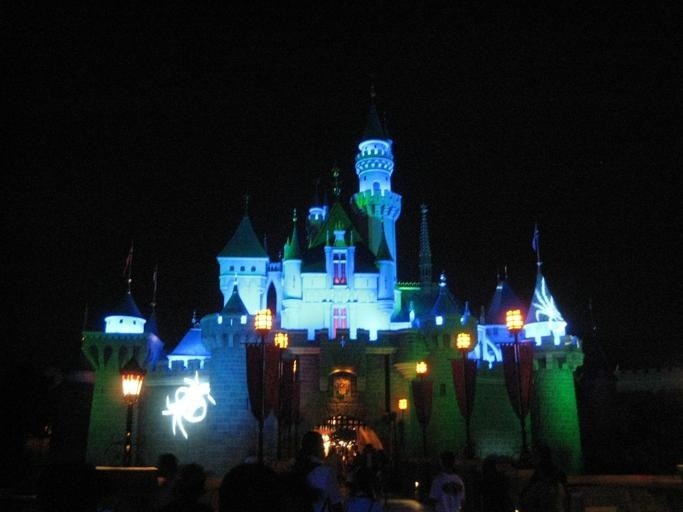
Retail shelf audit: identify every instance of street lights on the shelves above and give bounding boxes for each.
[119,351,147,468]
[456,331,473,441]
[415,360,428,456]
[505,308,528,449]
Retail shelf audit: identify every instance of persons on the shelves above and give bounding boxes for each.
[1,419,577,511]
